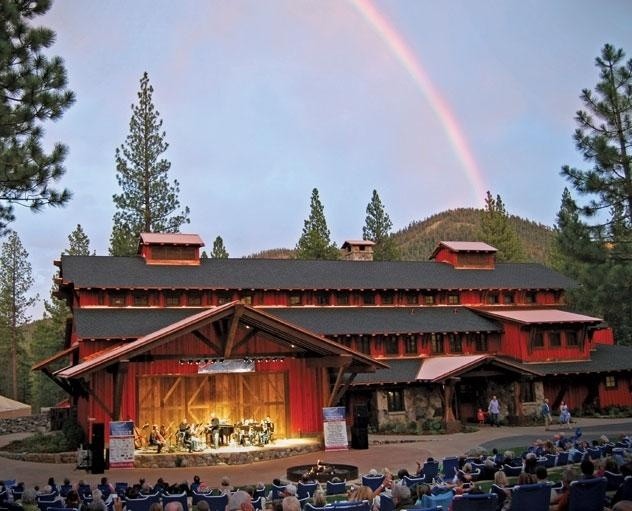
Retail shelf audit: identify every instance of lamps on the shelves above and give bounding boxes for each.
[177,358,224,364]
[242,355,286,363]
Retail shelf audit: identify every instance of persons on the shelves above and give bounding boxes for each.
[478,409,489,427]
[179,419,191,433]
[160,424,168,441]
[234,415,273,448]
[488,396,500,428]
[149,424,164,454]
[210,412,220,449]
[184,425,195,453]
[542,399,552,432]
[559,401,572,430]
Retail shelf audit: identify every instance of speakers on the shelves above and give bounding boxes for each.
[90,423,104,474]
[351,403,369,449]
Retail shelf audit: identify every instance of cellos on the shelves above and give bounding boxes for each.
[155,430,166,447]
[130,419,147,448]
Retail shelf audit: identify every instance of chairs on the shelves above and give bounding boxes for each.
[1,469,419,510]
[404,438,631,509]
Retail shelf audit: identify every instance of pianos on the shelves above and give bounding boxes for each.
[206,424,233,445]
